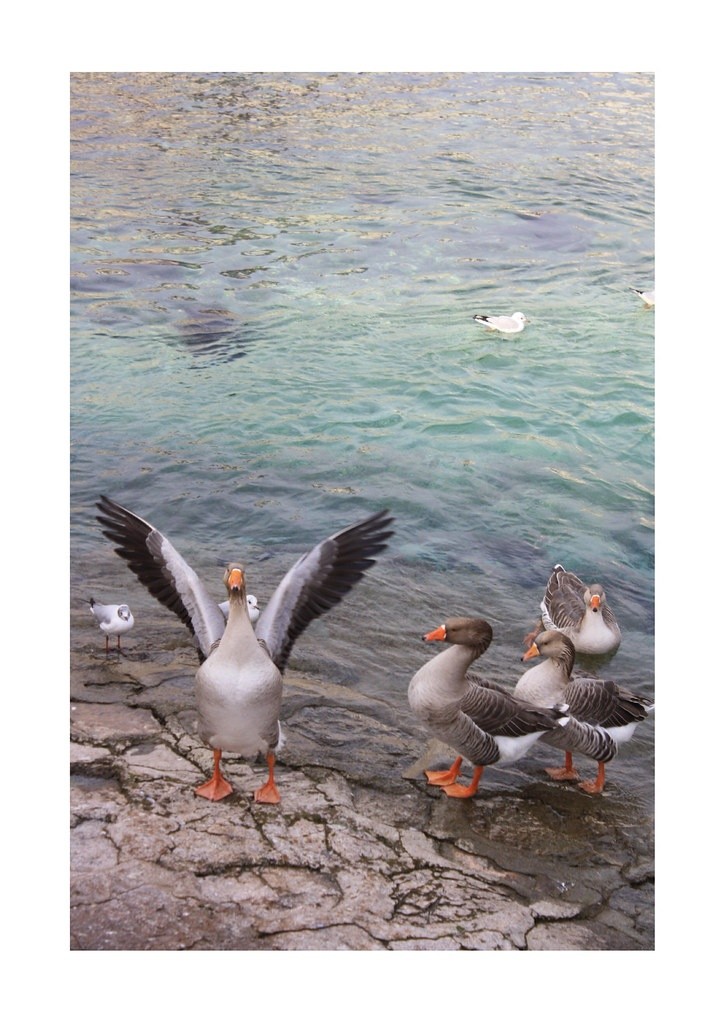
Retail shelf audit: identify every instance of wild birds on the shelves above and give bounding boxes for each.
[93,492,398,804]
[407,614,567,804]
[89,597,135,654]
[511,630,655,794]
[473,311,532,334]
[629,287,655,308]
[521,562,621,655]
